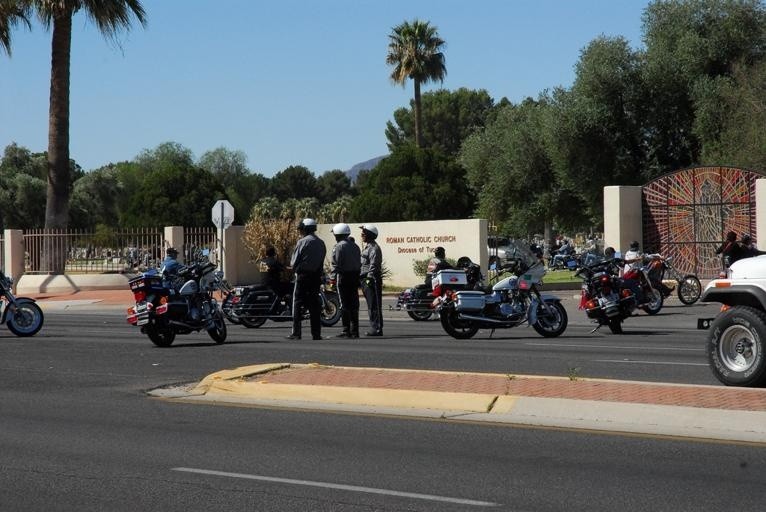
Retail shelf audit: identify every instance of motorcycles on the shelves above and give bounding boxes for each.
[621,266,663,317]
[574,260,638,333]
[644,253,703,305]
[0,270,43,336]
[398,258,483,320]
[127,249,229,346]
[431,239,568,339]
[546,249,602,269]
[234,272,343,327]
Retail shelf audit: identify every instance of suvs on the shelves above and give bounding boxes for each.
[696,256,765,388]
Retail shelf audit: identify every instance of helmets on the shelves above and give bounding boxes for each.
[166,248,179,256]
[266,246,276,256]
[358,224,378,239]
[629,241,640,251]
[605,247,615,254]
[741,233,752,246]
[297,218,317,233]
[329,223,351,236]
[435,247,445,253]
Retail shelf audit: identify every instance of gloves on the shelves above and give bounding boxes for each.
[330,284,338,293]
[366,278,374,285]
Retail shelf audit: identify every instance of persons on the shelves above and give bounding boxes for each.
[715,231,742,268]
[256,245,286,298]
[330,221,360,339]
[605,245,652,309]
[358,226,384,336]
[160,247,188,291]
[530,234,603,272]
[625,240,675,298]
[424,247,454,285]
[738,235,766,259]
[285,217,326,340]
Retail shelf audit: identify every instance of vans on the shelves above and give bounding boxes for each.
[487,237,516,264]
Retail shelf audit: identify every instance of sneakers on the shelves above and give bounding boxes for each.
[667,286,676,295]
[313,336,323,340]
[337,332,358,339]
[365,330,382,336]
[287,333,301,339]
